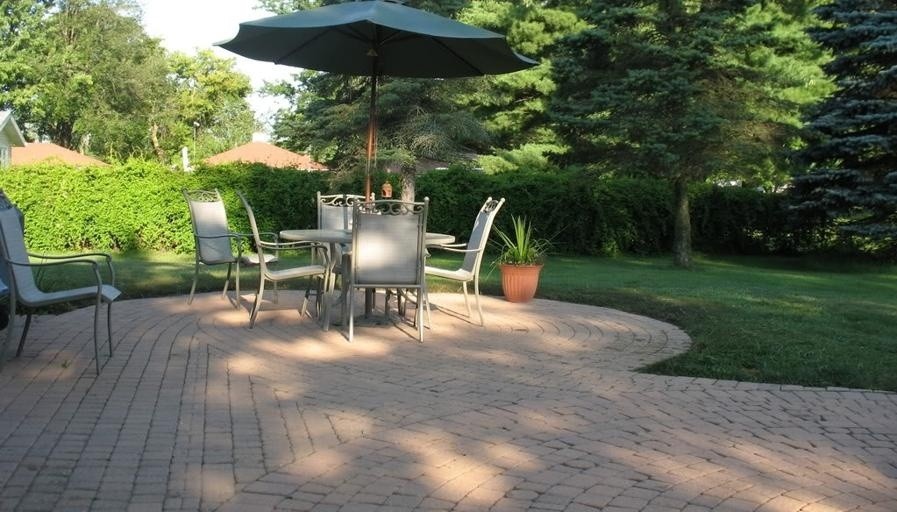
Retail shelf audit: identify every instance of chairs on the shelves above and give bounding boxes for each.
[0,208,121,375]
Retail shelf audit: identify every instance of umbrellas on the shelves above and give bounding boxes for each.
[210,1,543,318]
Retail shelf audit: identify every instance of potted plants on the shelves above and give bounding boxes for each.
[486,214,569,303]
